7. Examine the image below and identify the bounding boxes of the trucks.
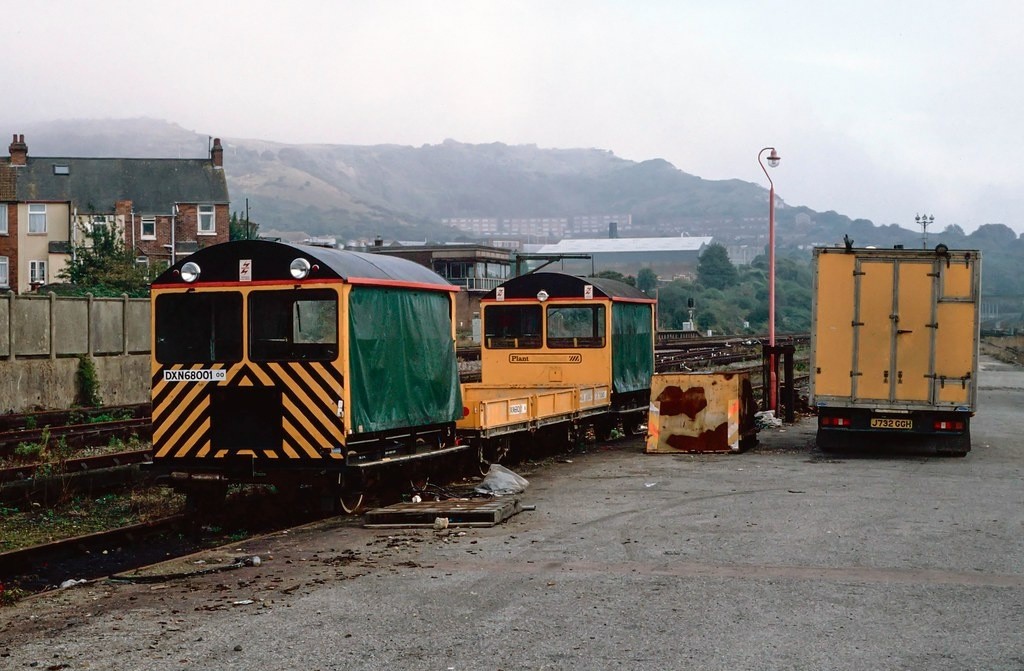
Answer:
[809,245,982,457]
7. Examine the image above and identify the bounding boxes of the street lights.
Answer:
[915,212,937,249]
[756,145,780,414]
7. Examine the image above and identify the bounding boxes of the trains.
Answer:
[144,238,659,516]
